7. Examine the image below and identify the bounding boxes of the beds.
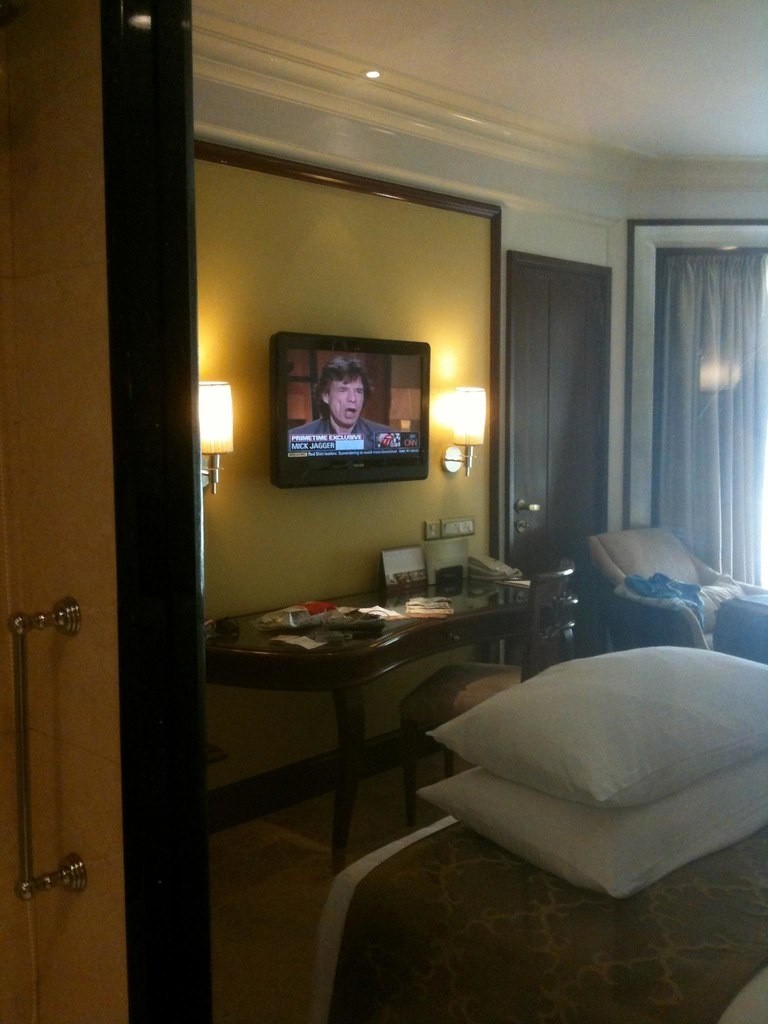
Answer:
[310,818,768,1024]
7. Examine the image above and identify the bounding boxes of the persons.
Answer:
[287,358,396,452]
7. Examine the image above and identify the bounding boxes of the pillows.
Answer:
[420,643,768,810]
[418,753,768,900]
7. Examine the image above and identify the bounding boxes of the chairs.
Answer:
[400,556,577,828]
[585,524,767,652]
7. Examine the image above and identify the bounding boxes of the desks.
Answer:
[206,572,578,877]
[712,593,768,665]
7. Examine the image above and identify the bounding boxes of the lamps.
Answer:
[442,387,487,477]
[199,381,234,495]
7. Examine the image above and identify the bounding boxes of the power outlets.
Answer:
[441,515,475,537]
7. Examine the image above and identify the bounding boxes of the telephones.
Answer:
[468,554,511,577]
